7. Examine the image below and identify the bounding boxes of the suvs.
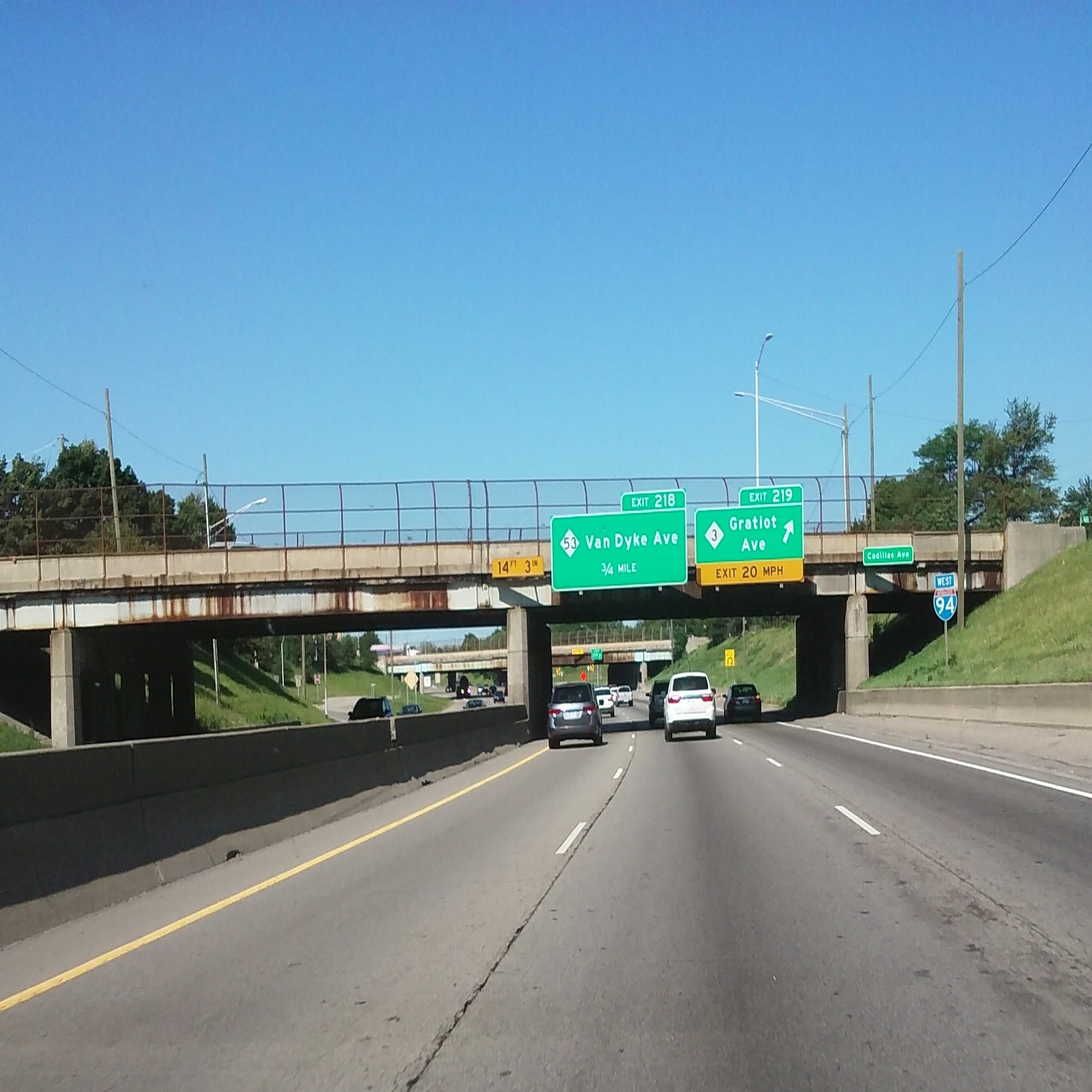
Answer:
[722,684,762,722]
[347,695,393,723]
[645,678,670,725]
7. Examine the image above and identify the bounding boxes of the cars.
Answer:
[396,704,424,718]
[457,686,472,699]
[444,687,453,693]
[463,700,487,711]
[475,684,506,704]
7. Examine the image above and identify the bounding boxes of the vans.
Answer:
[594,683,634,719]
[663,671,717,741]
[546,682,604,748]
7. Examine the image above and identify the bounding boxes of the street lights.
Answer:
[752,330,778,491]
[735,387,857,529]
[204,495,269,708]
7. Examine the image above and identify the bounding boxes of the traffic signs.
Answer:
[591,648,603,662]
[691,508,809,586]
